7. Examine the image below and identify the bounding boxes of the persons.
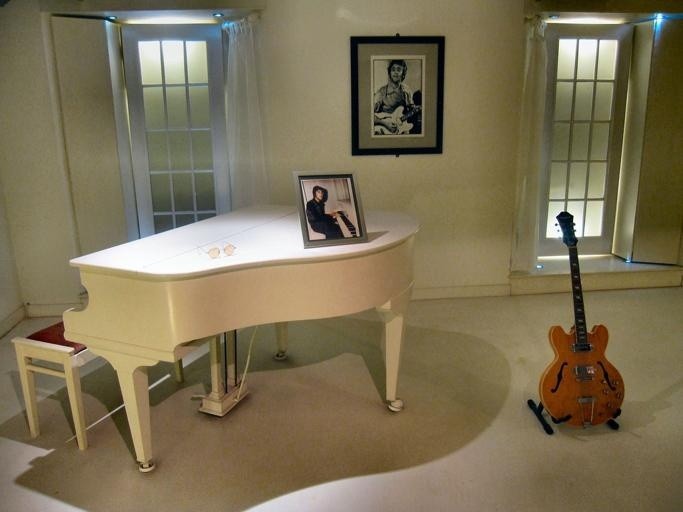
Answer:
[373,59,416,133]
[306,185,343,240]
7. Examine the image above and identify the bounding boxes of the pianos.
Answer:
[62,201,419,472]
[335,210,357,238]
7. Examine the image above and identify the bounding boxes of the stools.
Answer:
[11,321,184,451]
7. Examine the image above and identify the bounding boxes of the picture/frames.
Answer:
[350,35,446,156]
[292,168,369,250]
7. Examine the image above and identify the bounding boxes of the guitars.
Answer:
[538,211,623,428]
[374,106,420,135]
